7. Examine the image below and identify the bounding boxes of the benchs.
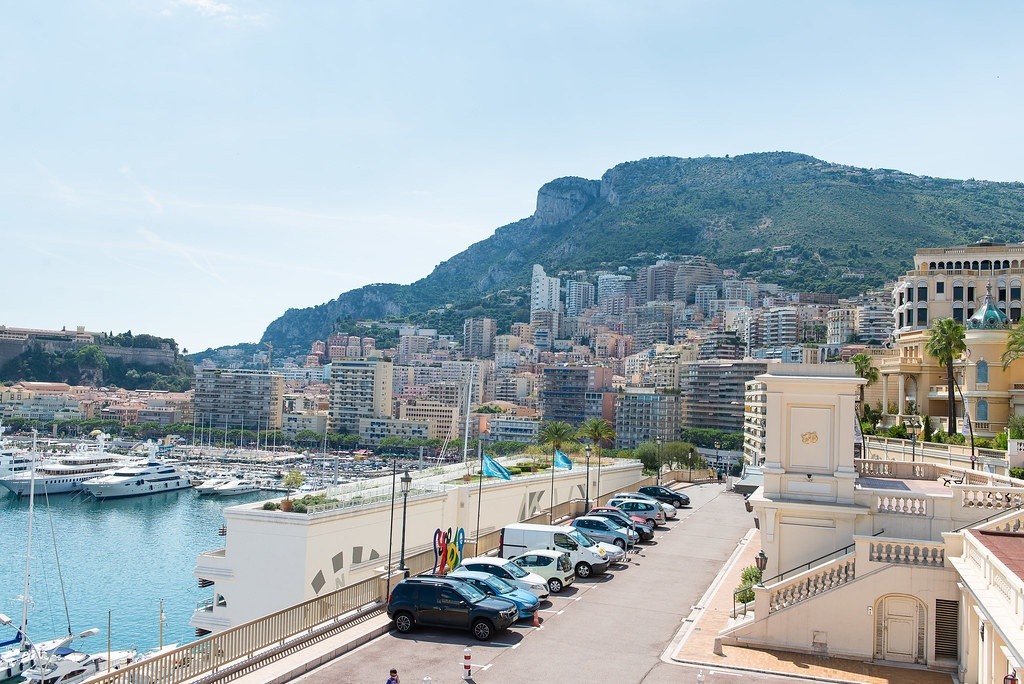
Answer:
[942,470,965,487]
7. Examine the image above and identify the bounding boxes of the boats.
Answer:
[0,434,139,496]
[195,476,261,496]
[81,439,194,498]
[173,413,429,491]
[0,447,54,478]
[0,429,179,684]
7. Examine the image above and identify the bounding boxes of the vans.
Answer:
[498,523,611,578]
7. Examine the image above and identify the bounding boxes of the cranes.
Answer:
[263,341,274,373]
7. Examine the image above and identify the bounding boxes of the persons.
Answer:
[385,668,400,684]
[709,467,715,484]
[716,466,724,485]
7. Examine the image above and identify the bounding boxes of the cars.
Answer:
[571,516,639,549]
[420,569,540,620]
[603,499,667,529]
[586,506,655,542]
[639,486,690,508]
[612,492,676,519]
[511,549,576,593]
[460,557,550,602]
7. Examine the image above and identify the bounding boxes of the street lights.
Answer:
[585,445,593,514]
[0,613,101,684]
[714,441,720,468]
[399,469,412,568]
[655,435,662,486]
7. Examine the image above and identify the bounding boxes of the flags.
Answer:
[554,448,572,470]
[483,453,512,480]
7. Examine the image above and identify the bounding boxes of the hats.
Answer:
[390,668,397,677]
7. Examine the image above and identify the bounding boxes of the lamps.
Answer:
[744,494,753,513]
[755,549,769,571]
[807,473,812,479]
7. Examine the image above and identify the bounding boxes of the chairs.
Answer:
[587,521,596,529]
[596,521,600,530]
[634,505,639,509]
[630,504,634,509]
[654,488,661,494]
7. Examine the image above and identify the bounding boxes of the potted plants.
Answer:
[540,443,552,463]
[279,470,306,512]
[463,457,475,484]
[525,444,542,472]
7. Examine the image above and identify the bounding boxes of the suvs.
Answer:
[387,574,519,641]
